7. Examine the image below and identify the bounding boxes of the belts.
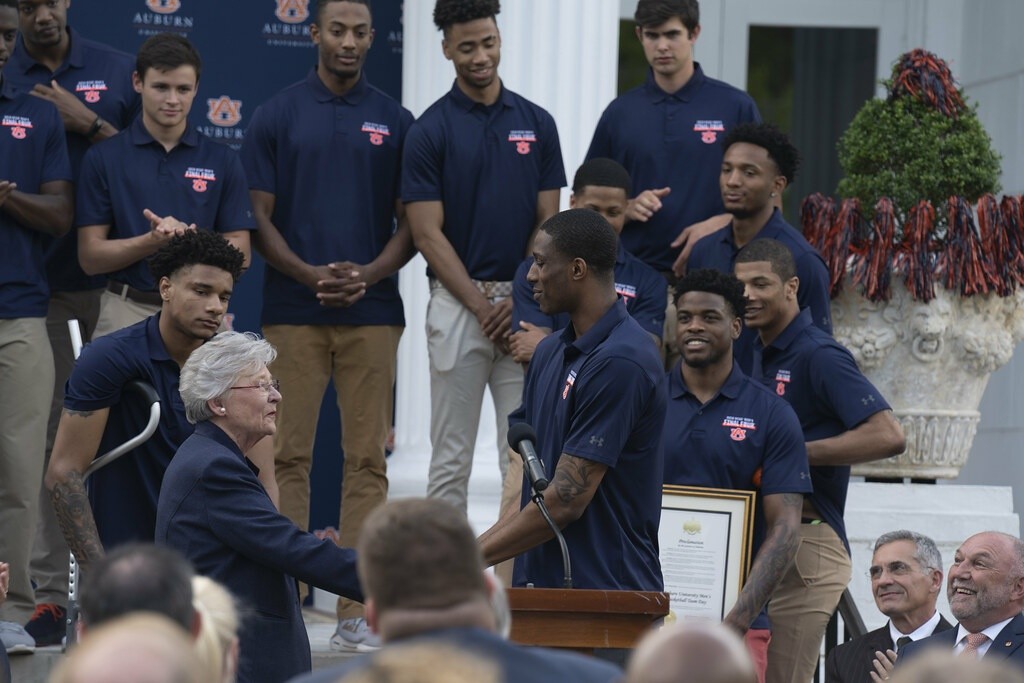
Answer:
[430,278,513,298]
[107,280,163,307]
[801,518,827,525]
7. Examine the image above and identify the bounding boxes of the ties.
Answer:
[896,636,914,655]
[958,633,990,661]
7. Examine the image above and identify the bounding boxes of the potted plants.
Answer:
[808,51,1024,477]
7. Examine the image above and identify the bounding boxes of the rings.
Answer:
[884,677,891,681]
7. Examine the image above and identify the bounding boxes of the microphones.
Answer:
[507,422,548,491]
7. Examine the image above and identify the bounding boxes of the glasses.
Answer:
[229,379,279,392]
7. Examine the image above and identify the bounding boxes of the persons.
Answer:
[0,0,833,650]
[823,530,956,683]
[894,531,1024,683]
[477,123,907,683]
[1,499,760,682]
[52,230,369,683]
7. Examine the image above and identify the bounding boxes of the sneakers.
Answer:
[329,617,382,652]
[0,621,36,653]
[25,604,67,652]
